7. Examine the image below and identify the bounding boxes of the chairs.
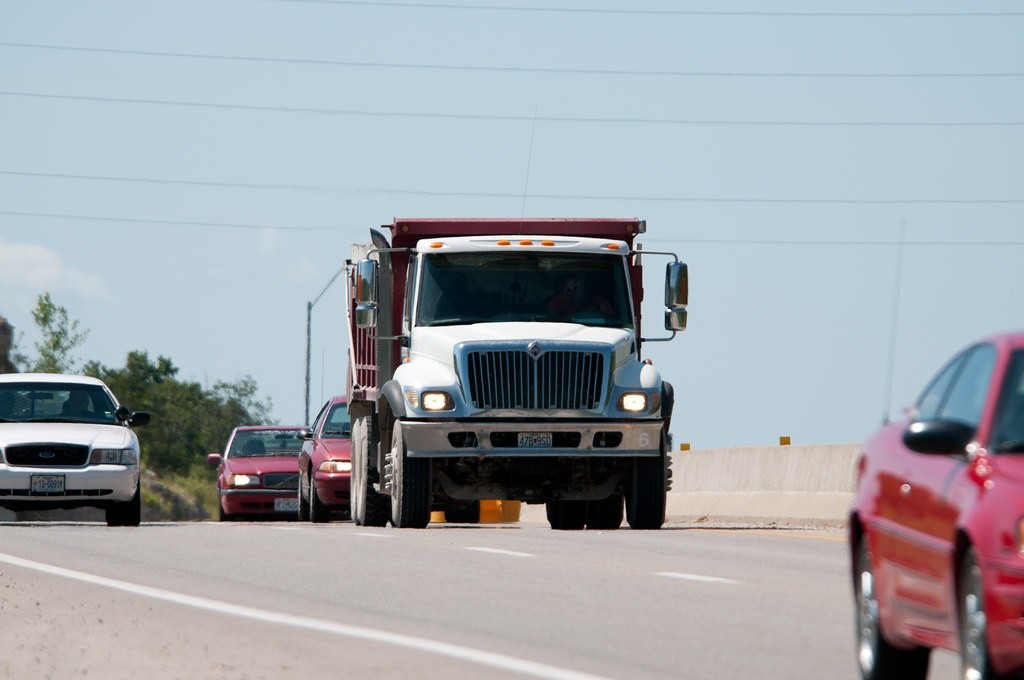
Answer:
[62,400,73,416]
[247,439,266,455]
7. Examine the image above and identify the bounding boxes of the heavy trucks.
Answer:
[344,216,689,529]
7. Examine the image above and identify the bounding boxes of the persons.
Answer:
[67,390,95,420]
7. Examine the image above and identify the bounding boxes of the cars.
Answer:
[208,426,314,521]
[846,335,1024,679]
[296,396,352,523]
[0,373,152,527]
